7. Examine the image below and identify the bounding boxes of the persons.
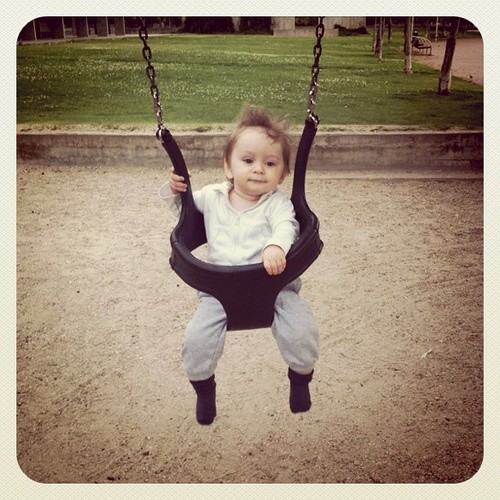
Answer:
[162,104,318,425]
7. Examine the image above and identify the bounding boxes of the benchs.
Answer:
[412,37,433,55]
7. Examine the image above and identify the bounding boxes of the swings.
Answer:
[136,17,324,331]
